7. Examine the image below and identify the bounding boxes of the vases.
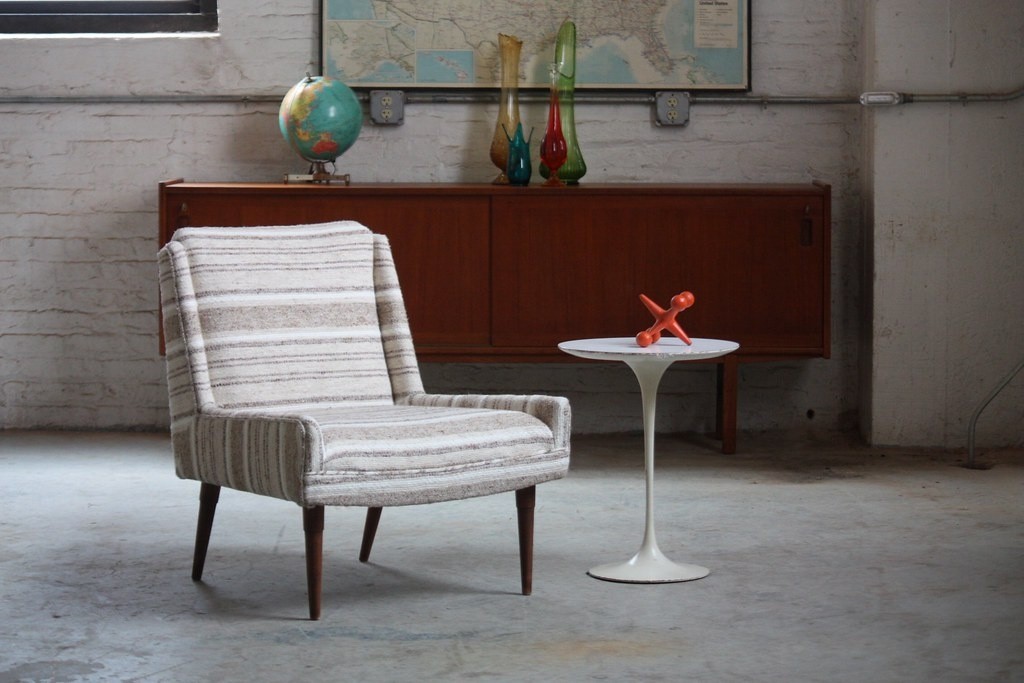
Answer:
[539,21,587,183]
[501,121,534,186]
[489,32,523,184]
[539,62,568,186]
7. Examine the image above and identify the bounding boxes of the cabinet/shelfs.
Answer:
[158,178,832,455]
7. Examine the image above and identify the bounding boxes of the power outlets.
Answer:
[368,90,404,126]
[654,91,691,126]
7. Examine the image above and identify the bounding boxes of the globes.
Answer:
[278,70,364,186]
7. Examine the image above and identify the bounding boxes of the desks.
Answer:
[557,338,740,584]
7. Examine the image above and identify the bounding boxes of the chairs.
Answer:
[157,221,571,620]
